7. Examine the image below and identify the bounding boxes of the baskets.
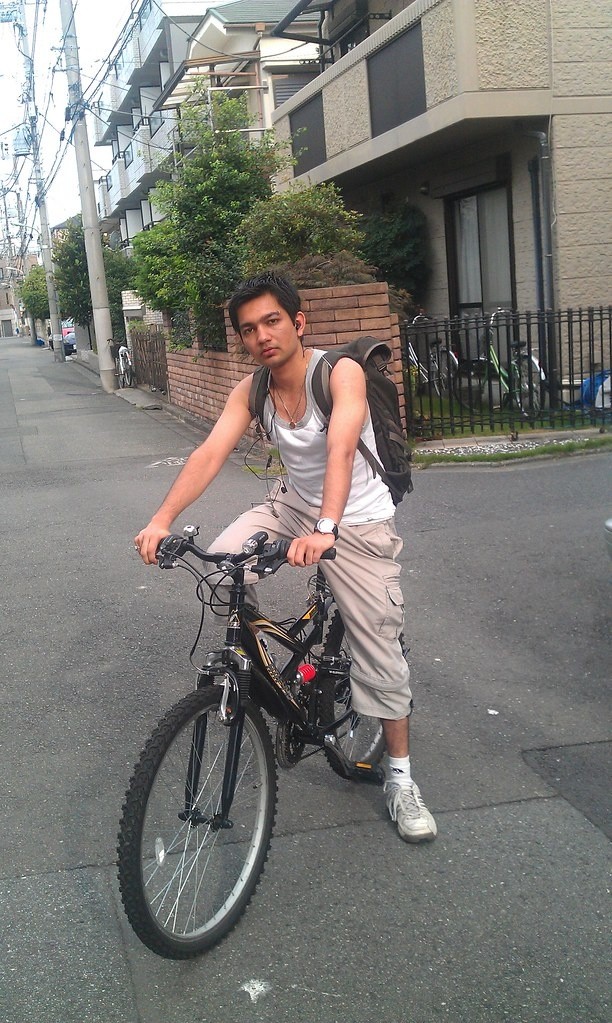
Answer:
[110,345,125,357]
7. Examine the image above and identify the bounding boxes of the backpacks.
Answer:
[248,336,415,507]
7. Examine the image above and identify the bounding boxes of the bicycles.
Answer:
[454,308,547,422]
[113,524,403,963]
[398,314,459,403]
[109,335,134,389]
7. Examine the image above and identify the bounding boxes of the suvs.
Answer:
[48,317,76,350]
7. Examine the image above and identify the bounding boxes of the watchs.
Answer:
[314,519,340,540]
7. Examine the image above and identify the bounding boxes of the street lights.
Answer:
[0,283,24,338]
[10,222,67,362]
[6,267,40,346]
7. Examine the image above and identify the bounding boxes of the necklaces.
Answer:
[275,354,307,429]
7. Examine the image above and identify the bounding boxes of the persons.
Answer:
[135,274,438,842]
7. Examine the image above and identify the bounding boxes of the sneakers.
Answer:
[383,781,437,843]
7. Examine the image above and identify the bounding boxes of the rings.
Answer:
[135,546,140,551]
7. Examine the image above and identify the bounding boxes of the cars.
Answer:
[63,331,76,356]
